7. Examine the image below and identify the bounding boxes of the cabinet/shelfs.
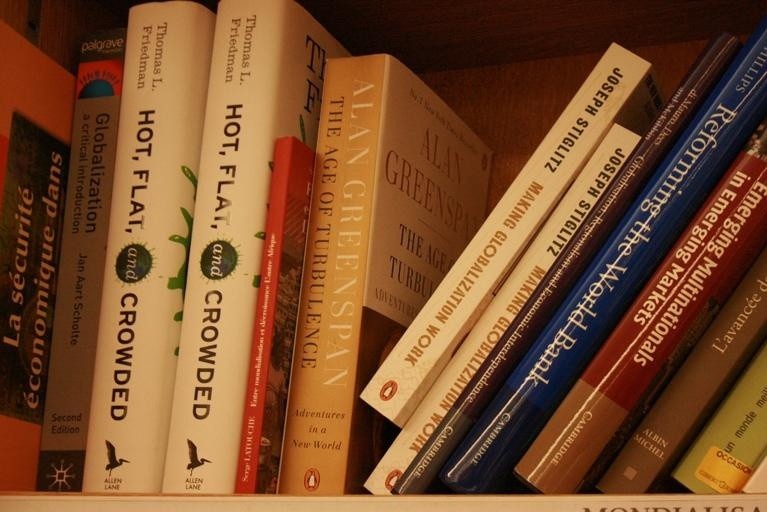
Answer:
[3,3,767,512]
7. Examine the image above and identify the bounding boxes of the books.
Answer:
[2,2,767,493]
